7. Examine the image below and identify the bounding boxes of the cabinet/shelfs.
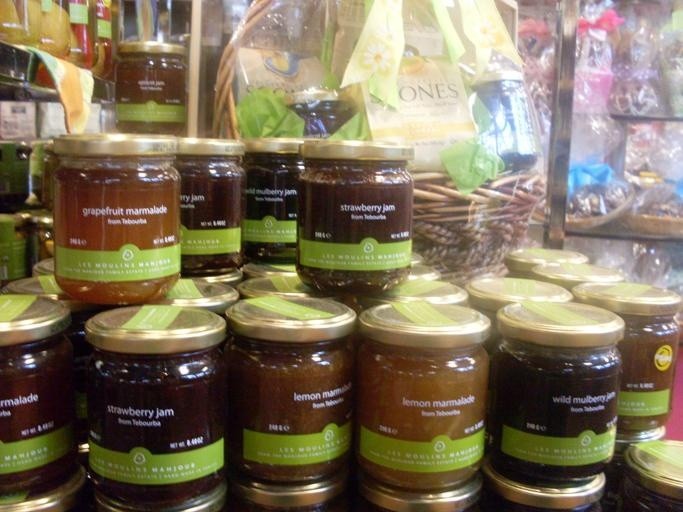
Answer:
[566,112,683,242]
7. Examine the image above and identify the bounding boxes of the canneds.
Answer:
[285,90,351,135]
[119,41,186,132]
[470,70,538,167]
[51,137,182,306]
[0,249,683,512]
[295,141,415,296]
[0,80,112,137]
[178,138,246,277]
[242,138,305,264]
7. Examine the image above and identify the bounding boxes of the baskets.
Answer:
[563,179,638,232]
[212,1,549,281]
[584,212,682,239]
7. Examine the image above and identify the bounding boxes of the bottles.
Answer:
[0,0,114,80]
